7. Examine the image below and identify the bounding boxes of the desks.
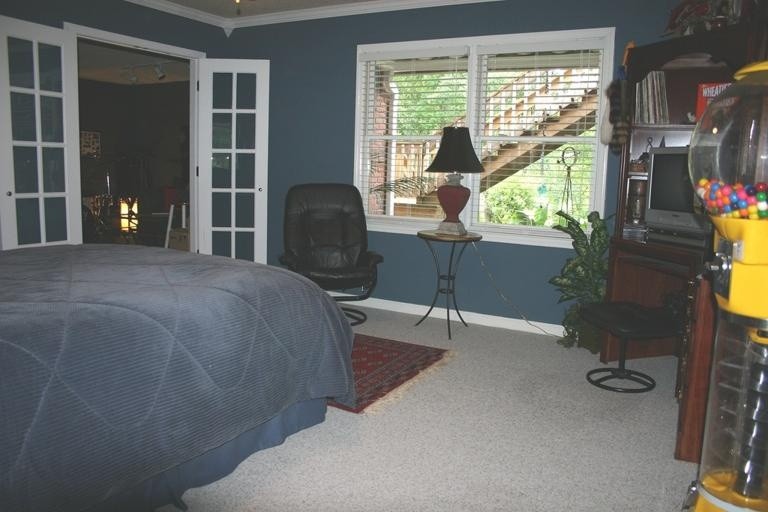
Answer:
[416,229,483,339]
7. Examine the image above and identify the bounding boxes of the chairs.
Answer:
[278,183,384,325]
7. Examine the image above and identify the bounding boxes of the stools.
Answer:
[580,302,667,392]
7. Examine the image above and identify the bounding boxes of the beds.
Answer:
[0,242,331,512]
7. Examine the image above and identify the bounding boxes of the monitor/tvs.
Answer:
[645,146,718,234]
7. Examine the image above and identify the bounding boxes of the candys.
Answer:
[695,178,768,219]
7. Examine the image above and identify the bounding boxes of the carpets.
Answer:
[328,332,448,414]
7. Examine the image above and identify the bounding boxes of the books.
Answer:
[634,70,669,124]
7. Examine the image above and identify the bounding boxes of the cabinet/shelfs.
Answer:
[605,11,768,466]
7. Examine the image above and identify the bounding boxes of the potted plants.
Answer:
[551,209,617,354]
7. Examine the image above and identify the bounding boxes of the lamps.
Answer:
[424,122,485,237]
